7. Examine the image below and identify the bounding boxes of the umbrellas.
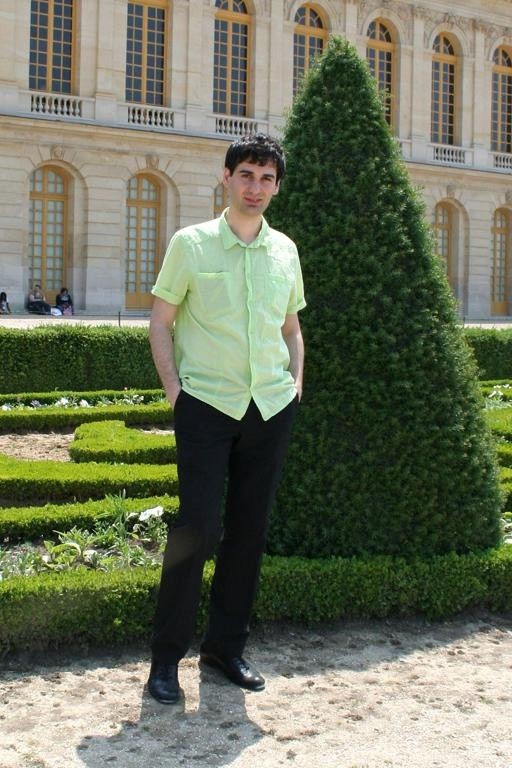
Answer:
[198,651,265,691]
[148,659,179,705]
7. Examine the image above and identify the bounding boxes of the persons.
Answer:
[28,285,50,315]
[148,132,307,705]
[56,288,72,313]
[0,292,11,315]
